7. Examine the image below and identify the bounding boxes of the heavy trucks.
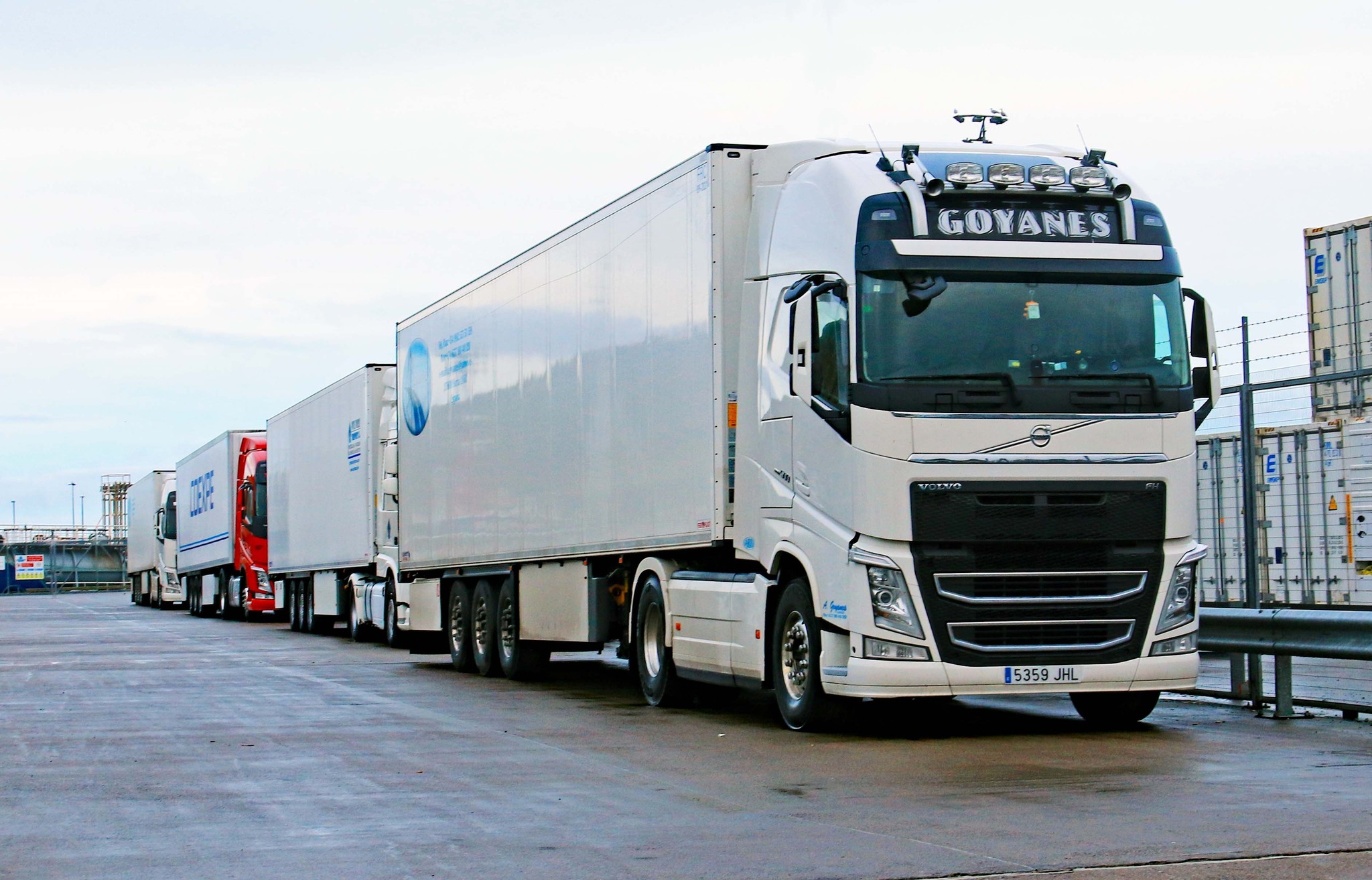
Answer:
[266,363,402,647]
[394,108,1221,730]
[175,430,290,622]
[127,470,188,610]
